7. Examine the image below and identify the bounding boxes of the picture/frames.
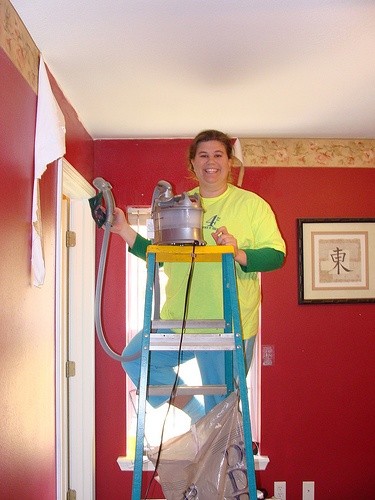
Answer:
[296,216,375,306]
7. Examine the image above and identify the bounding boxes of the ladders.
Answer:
[132,245,257,500]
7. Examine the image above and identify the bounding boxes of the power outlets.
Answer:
[274,481,286,500]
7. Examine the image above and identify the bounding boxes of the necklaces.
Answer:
[200,193,205,198]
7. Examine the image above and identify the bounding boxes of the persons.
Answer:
[101,130,286,427]
